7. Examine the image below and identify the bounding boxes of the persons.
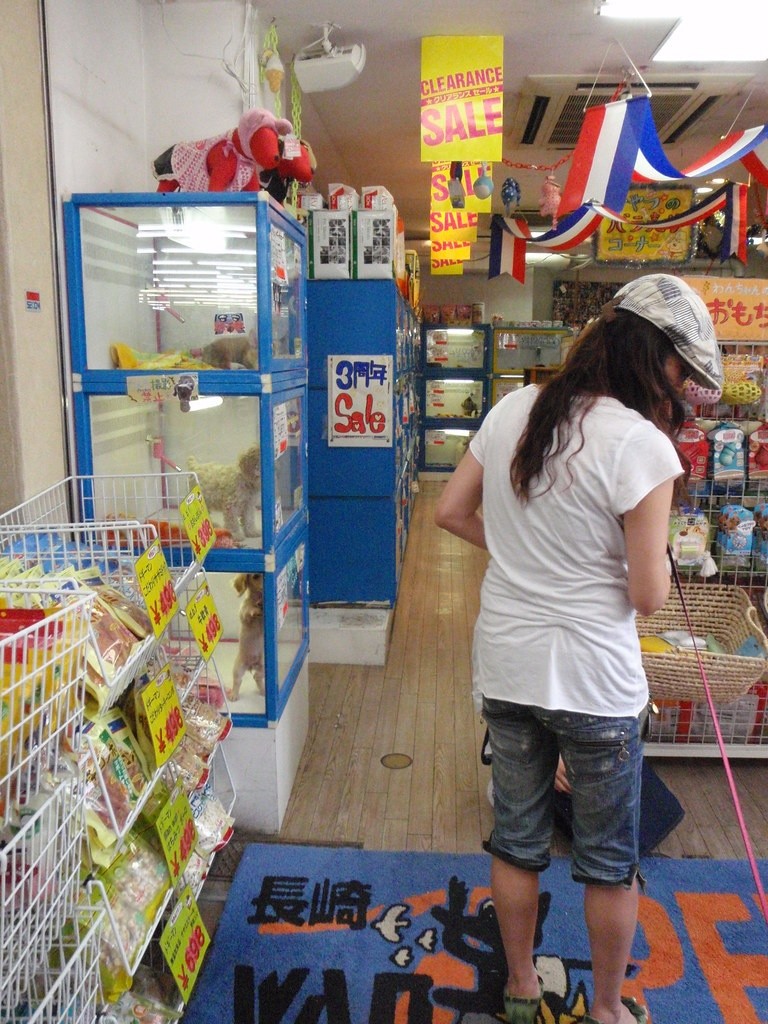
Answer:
[435,273,722,1024]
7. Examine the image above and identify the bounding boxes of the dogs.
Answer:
[186,443,262,544]
[228,573,267,703]
[198,326,257,369]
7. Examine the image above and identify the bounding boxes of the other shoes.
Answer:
[584,996,648,1024]
[503,971,544,1024]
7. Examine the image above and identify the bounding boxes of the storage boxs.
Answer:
[348,209,397,279]
[296,193,326,210]
[306,209,350,279]
[328,183,359,210]
[361,185,394,210]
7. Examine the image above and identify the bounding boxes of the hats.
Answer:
[600,273,724,389]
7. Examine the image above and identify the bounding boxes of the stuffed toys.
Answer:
[149,107,317,206]
[539,179,560,218]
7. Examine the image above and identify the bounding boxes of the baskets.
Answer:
[635,583,768,705]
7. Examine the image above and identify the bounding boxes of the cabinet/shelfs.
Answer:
[305,278,422,608]
[421,323,495,473]
[488,326,571,410]
[59,191,312,835]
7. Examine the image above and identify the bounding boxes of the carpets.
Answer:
[177,843,768,1024]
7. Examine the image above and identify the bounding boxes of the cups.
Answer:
[472,302,485,323]
